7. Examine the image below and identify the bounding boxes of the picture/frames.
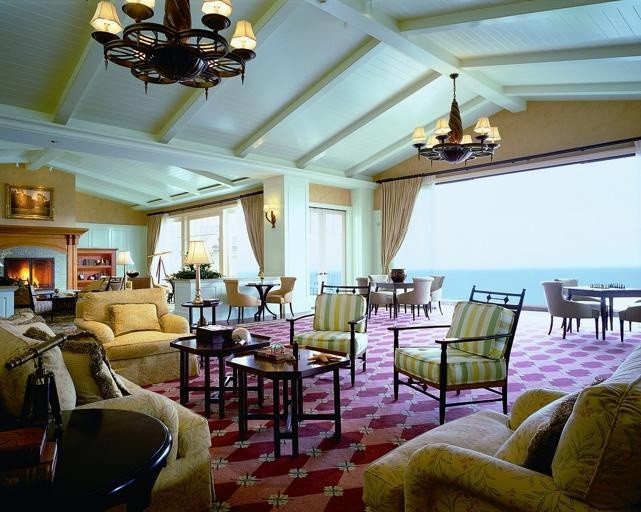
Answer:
[5,182,56,222]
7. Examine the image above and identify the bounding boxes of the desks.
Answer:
[2,407,173,512]
[182,301,222,333]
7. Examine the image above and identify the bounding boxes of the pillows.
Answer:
[107,302,163,337]
[493,371,614,474]
[22,325,133,405]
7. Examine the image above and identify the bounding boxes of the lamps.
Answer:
[410,72,503,168]
[89,0,259,101]
[115,249,135,290]
[184,241,213,305]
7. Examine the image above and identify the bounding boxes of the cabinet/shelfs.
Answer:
[77,248,119,283]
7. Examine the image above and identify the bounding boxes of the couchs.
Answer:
[2,308,218,511]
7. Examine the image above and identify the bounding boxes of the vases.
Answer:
[391,268,407,283]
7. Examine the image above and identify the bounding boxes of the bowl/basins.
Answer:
[231,328,251,342]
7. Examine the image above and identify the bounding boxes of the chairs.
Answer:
[541,277,641,344]
[388,285,526,425]
[74,286,199,387]
[223,277,297,322]
[362,346,641,510]
[284,280,372,388]
[355,274,446,323]
[151,275,173,304]
[28,276,111,321]
